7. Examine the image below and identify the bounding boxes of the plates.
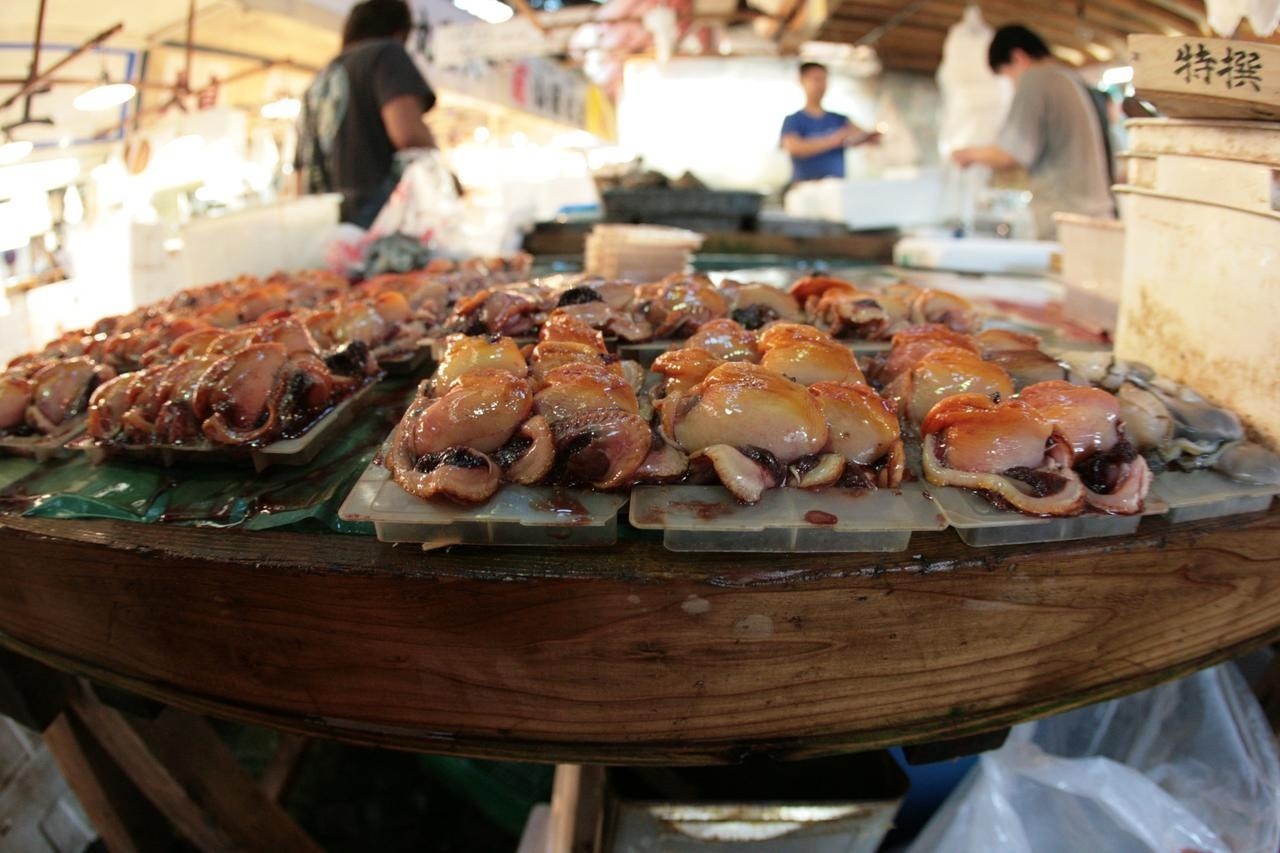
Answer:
[583,221,706,287]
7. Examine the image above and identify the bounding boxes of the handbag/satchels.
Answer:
[338,151,524,278]
[912,661,1280,851]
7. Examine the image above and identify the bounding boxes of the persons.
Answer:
[293,1,462,252]
[781,60,886,193]
[950,21,1123,245]
